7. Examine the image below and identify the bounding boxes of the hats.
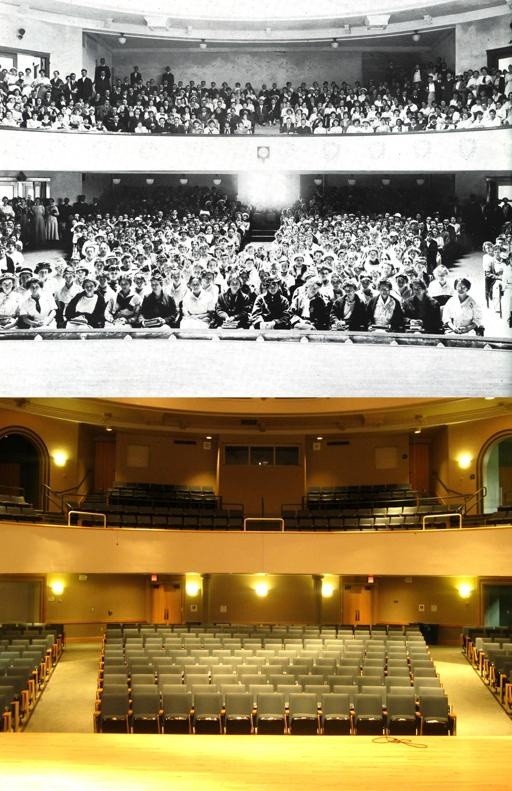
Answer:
[34,261,53,275]
[54,197,423,290]
[0,272,17,285]
[24,277,45,290]
[18,268,34,278]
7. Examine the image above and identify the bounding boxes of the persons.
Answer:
[0,180,512,344]
[0,56,511,136]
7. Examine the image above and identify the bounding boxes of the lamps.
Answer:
[117,33,127,45]
[412,30,421,43]
[200,39,207,51]
[331,38,339,49]
[113,174,223,189]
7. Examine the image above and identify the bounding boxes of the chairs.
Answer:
[0,623,512,736]
[0,481,511,531]
